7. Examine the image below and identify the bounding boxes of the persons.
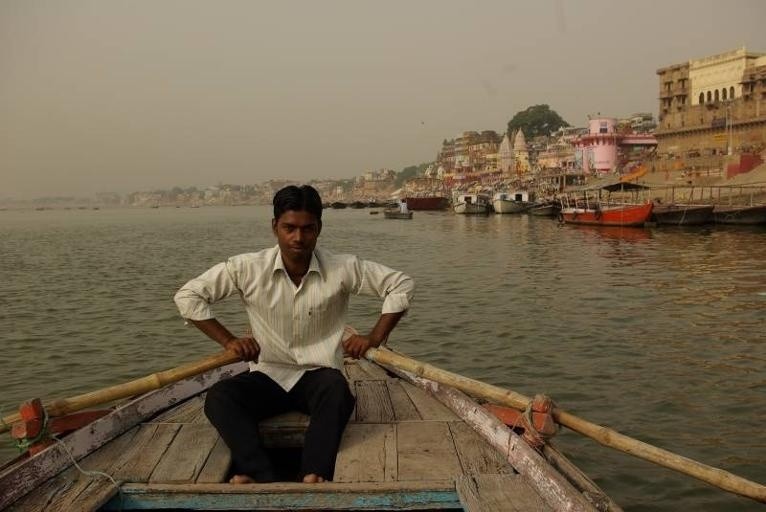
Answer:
[399,200,409,213]
[173,183,415,484]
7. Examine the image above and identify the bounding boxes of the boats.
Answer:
[402,195,451,210]
[0,322,766,512]
[707,198,766,226]
[559,192,656,229]
[528,199,562,220]
[654,200,715,227]
[383,204,414,220]
[454,195,492,213]
[320,201,397,209]
[492,196,535,213]
